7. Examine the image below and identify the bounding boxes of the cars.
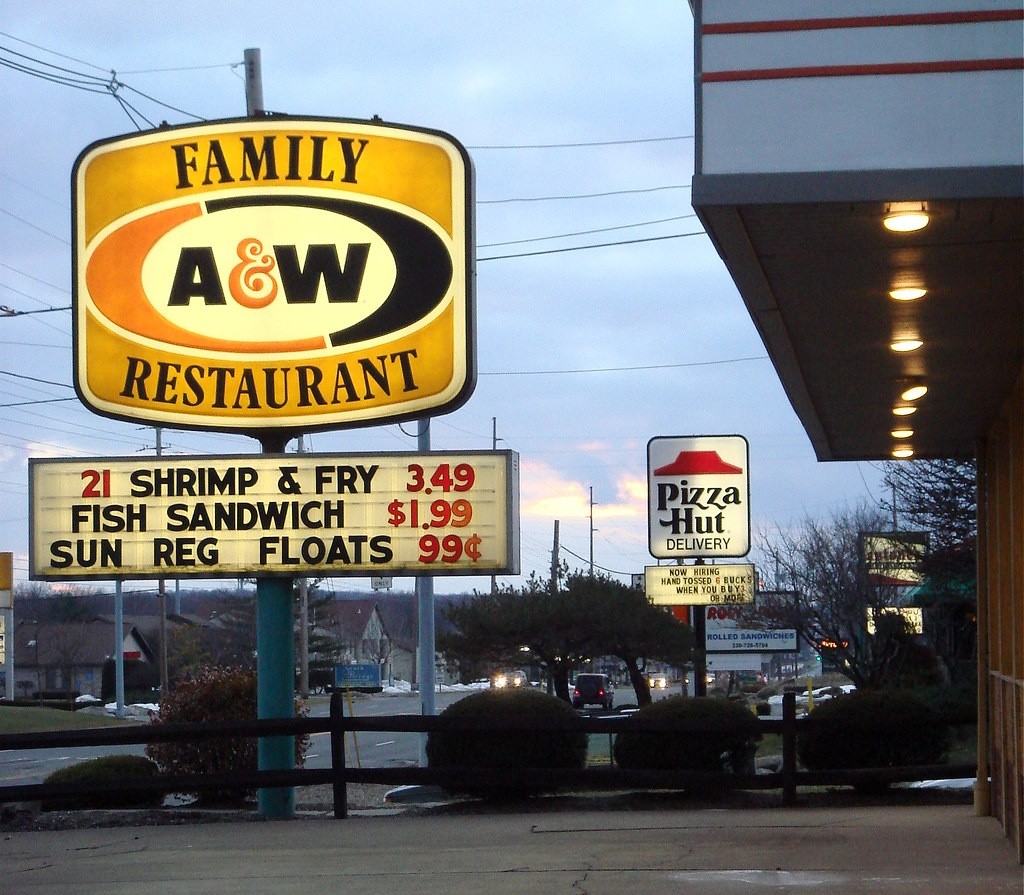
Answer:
[707,673,716,685]
[650,674,667,687]
[492,670,529,689]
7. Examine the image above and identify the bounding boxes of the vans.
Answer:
[573,673,614,711]
